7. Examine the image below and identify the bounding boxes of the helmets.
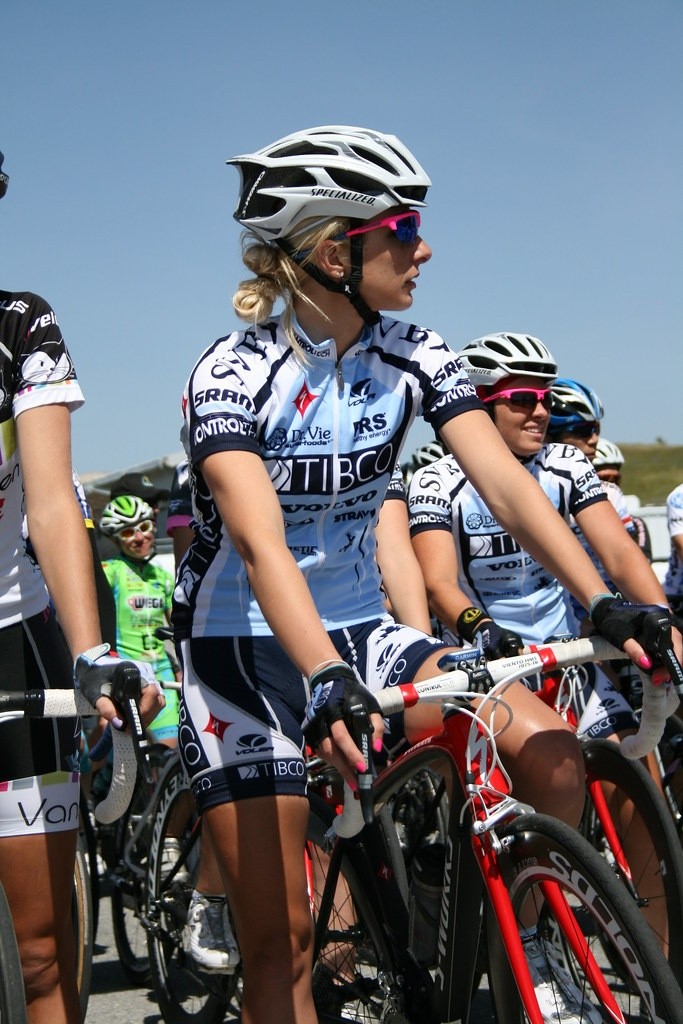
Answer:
[226,125,432,238]
[592,439,625,465]
[549,376,603,427]
[100,496,156,537]
[457,332,559,387]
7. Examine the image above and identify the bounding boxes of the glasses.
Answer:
[481,390,553,410]
[294,211,421,260]
[598,474,622,485]
[547,420,600,438]
[112,519,156,540]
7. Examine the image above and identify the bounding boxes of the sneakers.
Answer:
[521,933,592,1024]
[182,888,239,969]
[160,836,191,884]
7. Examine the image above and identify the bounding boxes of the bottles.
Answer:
[408,841,445,972]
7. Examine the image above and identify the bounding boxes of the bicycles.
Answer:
[66,631,682,1024]
[0,661,154,1023]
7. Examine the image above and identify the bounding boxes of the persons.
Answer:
[83,331,682,977]
[1,141,166,1024]
[166,123,673,1024]
